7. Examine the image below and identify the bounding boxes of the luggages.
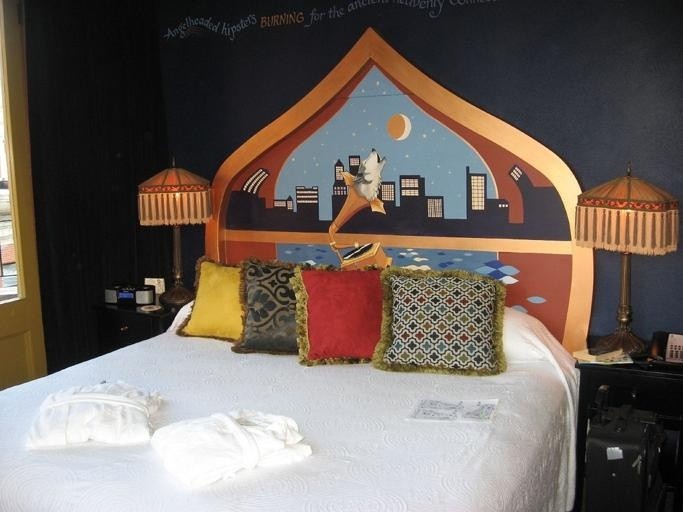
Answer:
[582,384,669,511]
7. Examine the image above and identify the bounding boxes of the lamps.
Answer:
[138,159,209,308]
[571,160,678,360]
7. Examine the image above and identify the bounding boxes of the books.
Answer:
[0,175,17,296]
[573,347,635,365]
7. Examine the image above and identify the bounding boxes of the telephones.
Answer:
[649,331,683,368]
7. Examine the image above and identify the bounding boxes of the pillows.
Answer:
[229,256,304,357]
[286,263,381,366]
[178,253,246,346]
[368,265,507,378]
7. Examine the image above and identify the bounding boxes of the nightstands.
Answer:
[93,291,168,339]
[568,360,679,508]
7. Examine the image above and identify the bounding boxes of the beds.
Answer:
[0,28,605,510]
